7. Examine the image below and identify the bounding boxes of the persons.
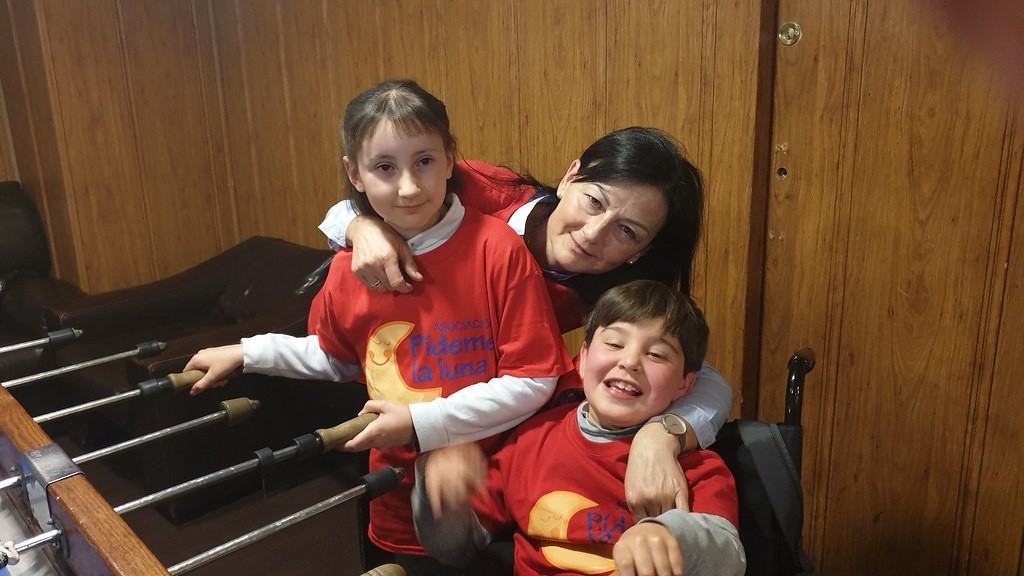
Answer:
[181,79,575,576]
[317,126,736,524]
[410,279,746,575]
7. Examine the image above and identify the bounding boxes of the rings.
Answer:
[371,280,382,288]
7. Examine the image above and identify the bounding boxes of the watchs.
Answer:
[644,413,686,454]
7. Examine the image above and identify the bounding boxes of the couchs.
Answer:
[0,181,92,441]
[42,235,369,523]
